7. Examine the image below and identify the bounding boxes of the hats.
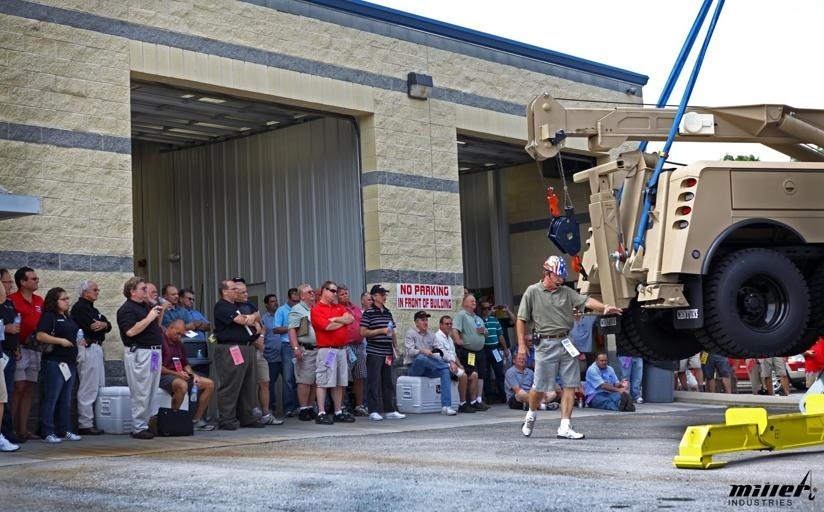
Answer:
[370,285,390,295]
[414,311,432,320]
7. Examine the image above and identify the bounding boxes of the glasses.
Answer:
[90,288,101,292]
[28,277,40,281]
[328,288,337,294]
[188,297,195,301]
[59,297,69,301]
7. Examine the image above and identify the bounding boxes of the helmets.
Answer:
[542,254,568,278]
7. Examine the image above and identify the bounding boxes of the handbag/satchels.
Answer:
[22,327,55,355]
[158,407,195,437]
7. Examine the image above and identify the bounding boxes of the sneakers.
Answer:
[557,424,585,440]
[260,414,284,425]
[131,429,155,439]
[298,405,370,425]
[521,412,537,438]
[458,399,492,414]
[441,406,457,416]
[192,416,237,432]
[541,401,560,411]
[385,410,407,420]
[239,418,265,428]
[367,411,383,421]
[9,426,104,444]
[0,434,21,453]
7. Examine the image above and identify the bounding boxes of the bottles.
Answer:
[11,314,21,334]
[475,319,481,333]
[189,383,198,402]
[158,296,172,311]
[76,328,84,346]
[196,349,203,359]
[577,397,582,408]
[386,320,393,337]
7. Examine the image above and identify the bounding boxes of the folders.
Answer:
[296,316,309,337]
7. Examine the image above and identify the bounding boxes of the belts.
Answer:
[317,346,348,350]
[122,345,163,350]
[88,340,104,346]
[538,331,571,340]
[296,341,317,349]
[216,341,256,347]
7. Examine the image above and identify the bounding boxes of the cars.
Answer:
[726,353,806,395]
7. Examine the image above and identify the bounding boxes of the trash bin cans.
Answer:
[641,360,676,403]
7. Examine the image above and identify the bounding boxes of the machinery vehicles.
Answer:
[525,92,824,364]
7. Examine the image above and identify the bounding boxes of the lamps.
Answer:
[406,70,433,101]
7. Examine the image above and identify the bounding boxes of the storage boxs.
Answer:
[394,374,463,416]
[94,385,192,436]
